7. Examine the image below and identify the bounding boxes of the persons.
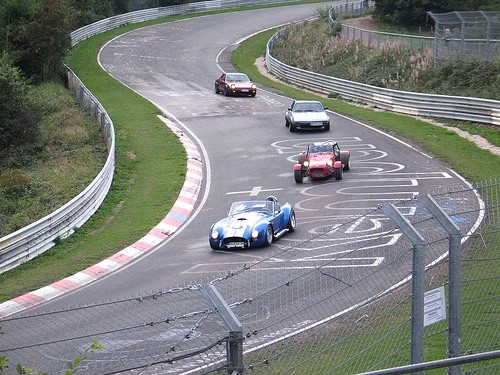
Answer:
[441,29,453,56]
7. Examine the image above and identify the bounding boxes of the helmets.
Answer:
[266,195,277,208]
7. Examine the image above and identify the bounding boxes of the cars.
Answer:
[284,99,331,133]
[214,72,257,97]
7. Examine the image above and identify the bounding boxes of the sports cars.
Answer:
[209,196,296,251]
[294,139,351,183]
